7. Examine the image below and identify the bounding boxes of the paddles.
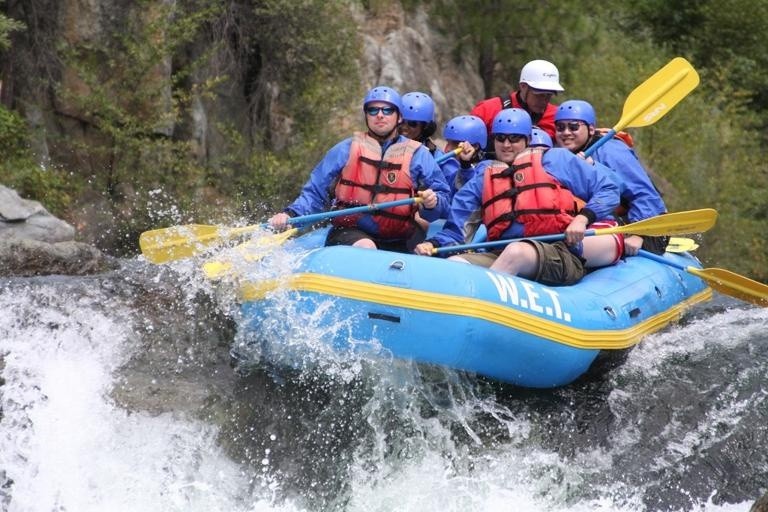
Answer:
[580,56,699,161]
[430,209,717,252]
[139,196,422,265]
[638,250,768,310]
[200,226,308,279]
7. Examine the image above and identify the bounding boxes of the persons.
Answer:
[268,85,451,255]
[415,108,621,286]
[400,59,670,265]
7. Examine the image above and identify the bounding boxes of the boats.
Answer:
[171,222,714,388]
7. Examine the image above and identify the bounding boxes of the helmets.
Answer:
[363,86,597,148]
[519,60,564,91]
